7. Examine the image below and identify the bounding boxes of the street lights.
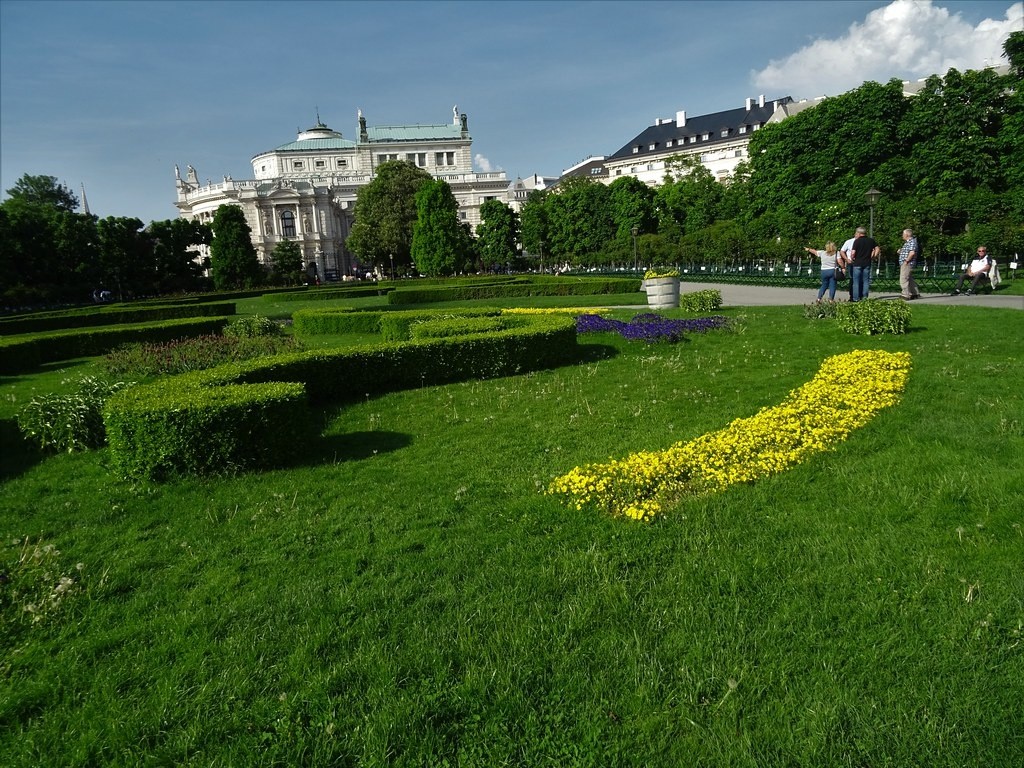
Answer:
[629,225,638,278]
[537,240,544,272]
[388,254,395,279]
[862,185,883,239]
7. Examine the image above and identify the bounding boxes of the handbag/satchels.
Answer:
[834,267,845,281]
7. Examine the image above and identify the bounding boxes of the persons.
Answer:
[951,244,992,296]
[851,227,880,302]
[840,227,856,302]
[897,229,918,301]
[804,241,846,303]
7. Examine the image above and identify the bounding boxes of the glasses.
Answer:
[978,250,985,252]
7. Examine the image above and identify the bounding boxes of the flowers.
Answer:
[644,267,680,280]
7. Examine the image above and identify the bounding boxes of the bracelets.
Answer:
[842,268,845,269]
[808,248,810,251]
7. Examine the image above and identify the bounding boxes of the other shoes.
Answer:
[951,290,960,296]
[815,299,821,306]
[964,290,972,296]
[828,299,833,305]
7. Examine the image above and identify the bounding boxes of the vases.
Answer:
[646,277,680,309]
